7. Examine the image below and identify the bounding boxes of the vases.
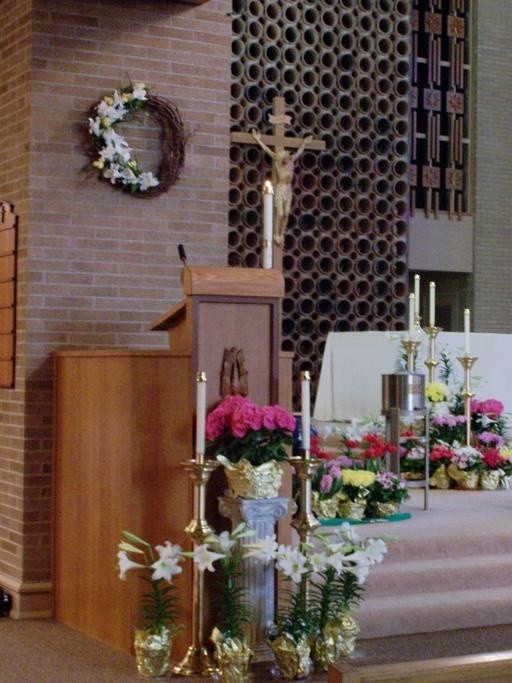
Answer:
[215,454,285,500]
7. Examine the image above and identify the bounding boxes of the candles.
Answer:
[408,274,471,355]
[195,371,207,455]
[261,181,274,269]
[301,370,310,450]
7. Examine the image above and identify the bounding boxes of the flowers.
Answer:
[205,395,297,466]
[310,335,512,519]
[85,81,185,199]
